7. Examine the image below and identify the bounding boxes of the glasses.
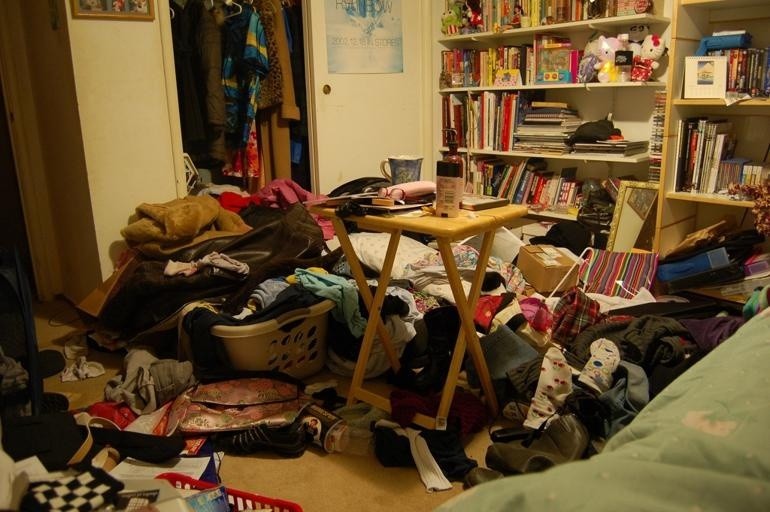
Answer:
[377,187,408,205]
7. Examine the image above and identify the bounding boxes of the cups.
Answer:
[379,153,424,187]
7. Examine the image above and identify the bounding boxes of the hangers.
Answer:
[208,1,292,19]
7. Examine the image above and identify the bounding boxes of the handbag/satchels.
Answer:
[575,242,661,305]
[656,213,767,294]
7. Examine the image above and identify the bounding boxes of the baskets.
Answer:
[145,471,303,512]
[211,298,337,380]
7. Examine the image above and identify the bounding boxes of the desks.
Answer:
[306,199,528,430]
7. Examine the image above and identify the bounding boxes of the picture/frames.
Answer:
[70,1,155,20]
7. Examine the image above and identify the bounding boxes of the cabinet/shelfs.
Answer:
[436,0,770,305]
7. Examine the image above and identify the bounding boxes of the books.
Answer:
[300,186,379,202]
[355,198,433,213]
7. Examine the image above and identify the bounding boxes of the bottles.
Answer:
[435,158,465,218]
